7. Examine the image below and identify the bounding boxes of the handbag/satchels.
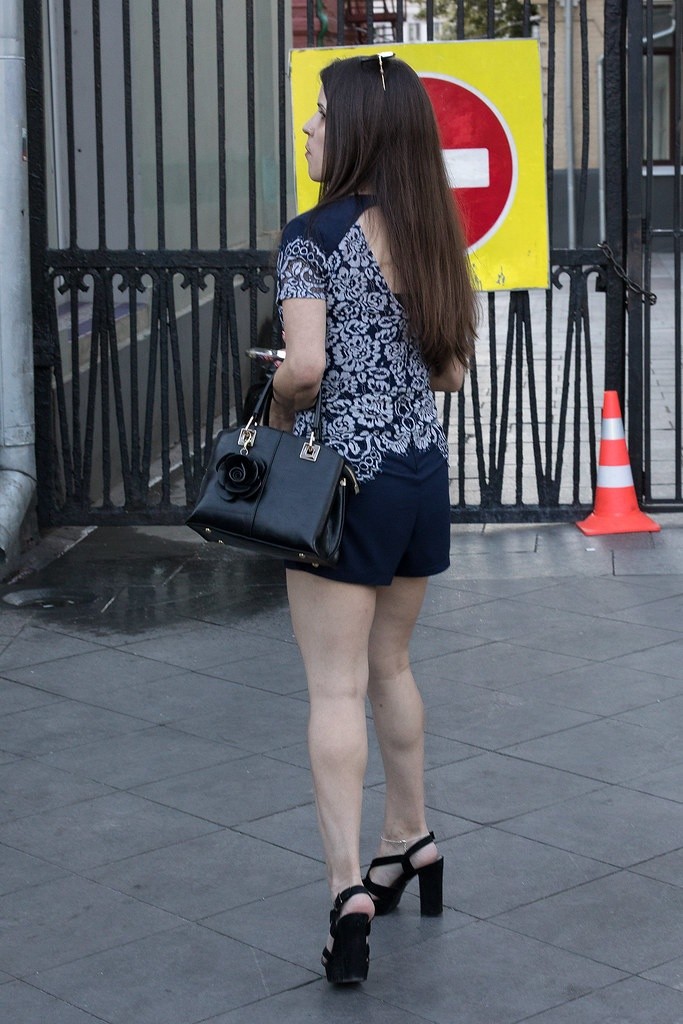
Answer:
[184,373,359,570]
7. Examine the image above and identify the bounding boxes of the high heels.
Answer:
[361,831,444,916]
[321,885,376,983]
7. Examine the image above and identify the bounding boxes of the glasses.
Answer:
[377,51,394,92]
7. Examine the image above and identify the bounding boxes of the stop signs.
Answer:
[282,36,553,294]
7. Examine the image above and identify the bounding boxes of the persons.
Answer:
[266,55,478,984]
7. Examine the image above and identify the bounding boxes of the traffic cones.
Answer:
[573,390,663,539]
